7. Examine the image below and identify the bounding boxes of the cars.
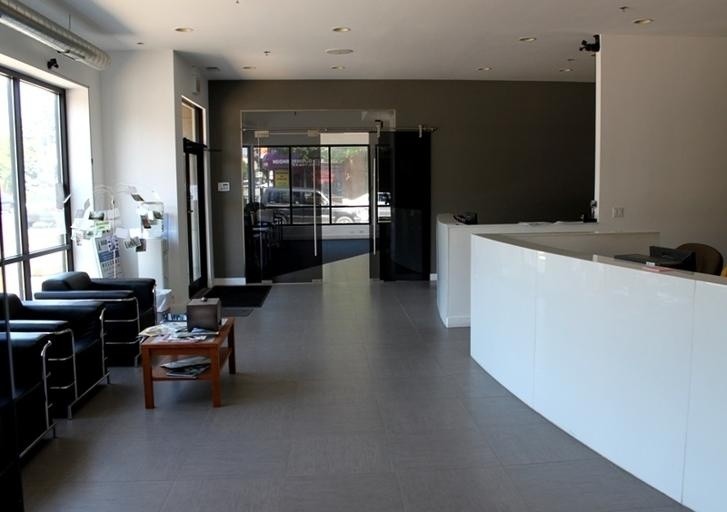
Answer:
[350,192,391,221]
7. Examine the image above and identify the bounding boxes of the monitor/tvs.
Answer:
[649,245,696,271]
[464,211,478,224]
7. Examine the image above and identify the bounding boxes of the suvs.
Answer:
[259,188,359,224]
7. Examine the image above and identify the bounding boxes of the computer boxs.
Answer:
[614,254,682,270]
[454,214,470,224]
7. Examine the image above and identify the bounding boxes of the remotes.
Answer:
[192,331,221,337]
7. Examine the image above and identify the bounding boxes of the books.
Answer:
[135,318,227,380]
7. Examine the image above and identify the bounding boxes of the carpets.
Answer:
[204,284,273,308]
[222,308,253,317]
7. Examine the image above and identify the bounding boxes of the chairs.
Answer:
[0,332,57,467]
[676,242,723,276]
[33,271,157,368]
[249,208,283,265]
[0,292,111,420]
